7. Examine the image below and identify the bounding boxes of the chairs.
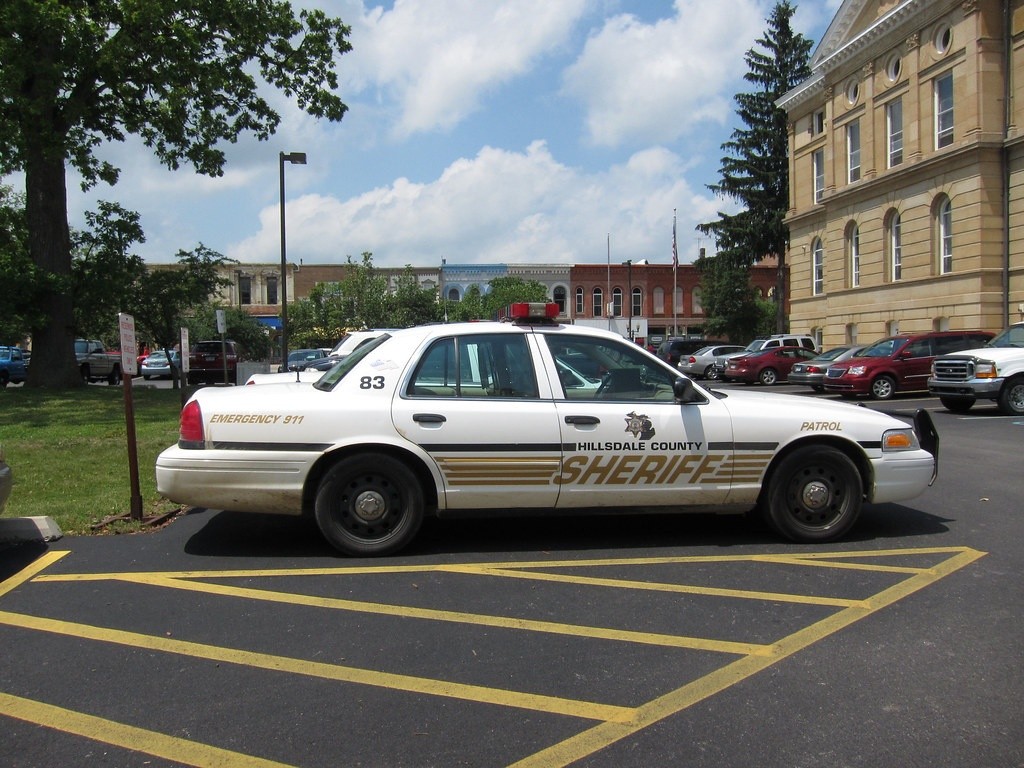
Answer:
[503,342,538,397]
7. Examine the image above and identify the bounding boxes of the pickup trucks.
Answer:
[73,338,123,386]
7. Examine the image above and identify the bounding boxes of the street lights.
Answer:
[621,260,633,340]
[233,268,249,342]
[278,151,309,374]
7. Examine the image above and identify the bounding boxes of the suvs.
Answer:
[653,335,739,370]
[926,320,1024,417]
[711,333,820,380]
[822,329,1008,401]
[0,344,32,387]
[185,340,243,386]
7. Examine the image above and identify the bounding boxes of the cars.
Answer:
[724,345,821,387]
[677,344,753,380]
[787,343,893,393]
[141,349,183,381]
[150,302,940,560]
[242,325,650,390]
